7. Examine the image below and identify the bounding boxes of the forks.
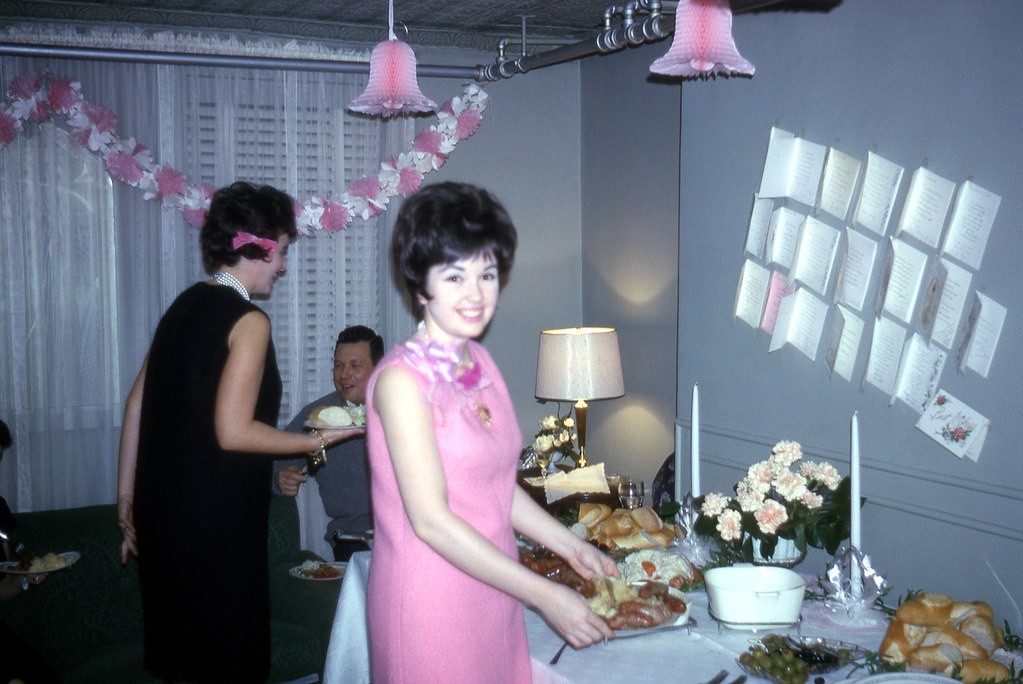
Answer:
[0,530,33,560]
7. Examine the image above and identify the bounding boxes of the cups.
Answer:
[619,479,646,510]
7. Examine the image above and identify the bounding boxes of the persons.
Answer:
[367,181,619,684]
[117,184,364,684]
[272,326,384,562]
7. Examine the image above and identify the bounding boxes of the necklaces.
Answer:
[209,272,250,301]
[418,321,493,426]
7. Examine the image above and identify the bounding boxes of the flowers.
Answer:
[0,69,489,251]
[532,415,581,464]
[690,441,867,562]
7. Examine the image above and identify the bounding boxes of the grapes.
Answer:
[740,636,850,684]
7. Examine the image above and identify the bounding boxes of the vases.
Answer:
[751,535,800,564]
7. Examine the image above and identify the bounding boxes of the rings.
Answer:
[121,527,129,532]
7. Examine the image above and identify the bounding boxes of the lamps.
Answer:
[347,0,437,116]
[535,326,626,466]
[692,382,700,497]
[850,411,861,593]
[650,0,755,76]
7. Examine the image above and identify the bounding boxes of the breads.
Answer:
[568,504,677,550]
[314,564,340,578]
[309,404,353,428]
[880,592,1011,684]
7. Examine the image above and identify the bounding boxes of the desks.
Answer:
[323,538,1023,684]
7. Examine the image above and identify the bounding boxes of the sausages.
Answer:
[602,582,688,628]
[519,544,595,596]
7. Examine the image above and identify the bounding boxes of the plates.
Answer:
[303,420,369,430]
[0,552,81,574]
[289,561,348,580]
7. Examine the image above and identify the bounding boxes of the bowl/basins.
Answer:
[705,567,806,631]
[854,673,965,684]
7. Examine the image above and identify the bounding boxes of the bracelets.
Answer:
[308,429,327,465]
